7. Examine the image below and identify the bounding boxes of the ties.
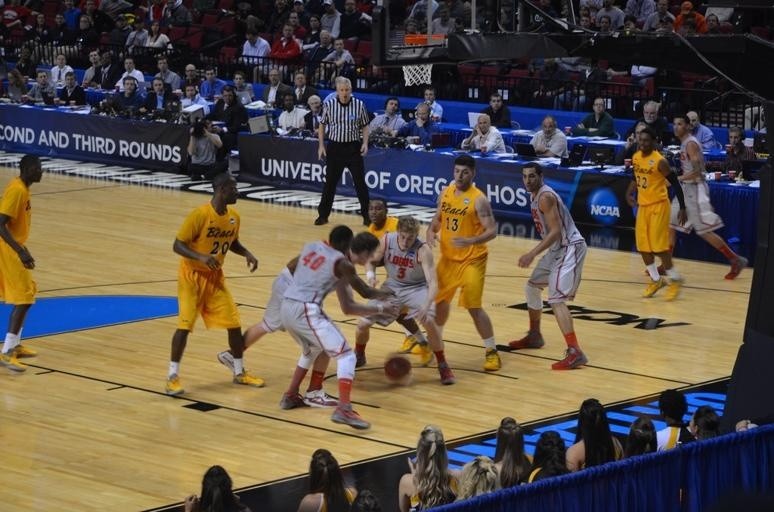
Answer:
[58,70,61,80]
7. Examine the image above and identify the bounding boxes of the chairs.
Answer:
[159,1,374,74]
[456,62,654,108]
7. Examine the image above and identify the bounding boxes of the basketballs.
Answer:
[385,358,411,383]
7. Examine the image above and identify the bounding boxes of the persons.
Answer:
[399,417,573,511]
[644,112,748,280]
[186,464,251,511]
[162,172,267,396]
[2,1,774,228]
[218,156,593,429]
[625,130,690,304]
[656,389,698,452]
[299,449,384,511]
[691,405,761,441]
[621,416,658,458]
[566,398,624,471]
[0,155,44,372]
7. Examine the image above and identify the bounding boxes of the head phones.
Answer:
[414,103,434,119]
[123,76,139,89]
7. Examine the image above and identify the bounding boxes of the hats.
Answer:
[324,0,333,5]
[680,1,692,15]
[293,0,303,4]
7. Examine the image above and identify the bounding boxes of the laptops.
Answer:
[513,142,539,161]
[40,91,54,105]
[424,134,451,150]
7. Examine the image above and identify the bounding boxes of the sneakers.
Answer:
[364,218,371,226]
[315,216,328,225]
[725,256,748,279]
[643,264,681,301]
[0,348,27,372]
[7,344,38,358]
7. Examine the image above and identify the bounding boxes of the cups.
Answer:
[83,81,88,89]
[53,98,58,107]
[115,85,120,93]
[729,170,736,181]
[725,144,731,154]
[481,146,486,155]
[624,158,632,170]
[564,126,572,136]
[715,171,722,181]
[407,135,420,144]
